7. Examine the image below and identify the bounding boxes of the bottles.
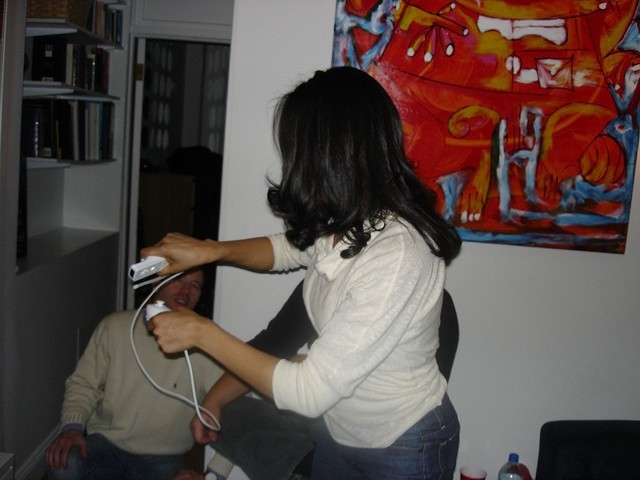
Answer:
[85,53,96,90]
[499,453,524,480]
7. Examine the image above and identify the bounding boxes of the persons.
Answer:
[42,269,224,480]
[138,67,462,480]
[190,277,460,480]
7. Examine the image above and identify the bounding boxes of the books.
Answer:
[91,2,124,43]
[33,36,110,91]
[28,101,114,161]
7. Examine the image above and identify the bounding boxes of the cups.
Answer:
[460,466,486,480]
[24,105,44,157]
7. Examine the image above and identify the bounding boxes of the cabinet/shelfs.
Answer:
[17,0,129,170]
